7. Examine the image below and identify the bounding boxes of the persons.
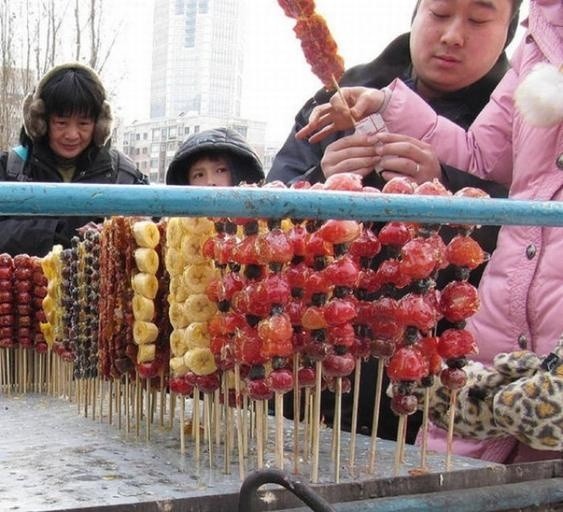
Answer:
[163,127,266,189]
[266,0,520,446]
[293,0,562,461]
[0,62,150,260]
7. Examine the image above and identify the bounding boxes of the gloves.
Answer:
[417,360,510,440]
[493,335,563,451]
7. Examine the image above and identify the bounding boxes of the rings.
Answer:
[415,164,421,176]
